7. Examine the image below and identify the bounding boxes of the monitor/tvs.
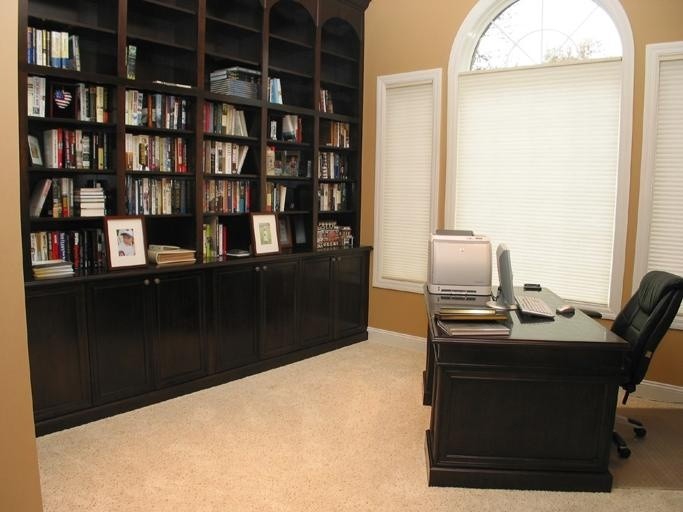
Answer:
[485,241,519,312]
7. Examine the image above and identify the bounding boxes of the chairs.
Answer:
[578,270,683,458]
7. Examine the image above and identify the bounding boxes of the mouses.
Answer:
[556,304,575,315]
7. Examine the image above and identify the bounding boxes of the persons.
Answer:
[117,229,135,257]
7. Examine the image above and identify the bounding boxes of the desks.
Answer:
[421,282,629,493]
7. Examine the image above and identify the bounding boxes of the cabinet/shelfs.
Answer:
[17,1,371,438]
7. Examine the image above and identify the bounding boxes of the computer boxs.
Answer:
[427,234,492,296]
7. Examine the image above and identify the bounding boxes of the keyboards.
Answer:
[515,294,556,319]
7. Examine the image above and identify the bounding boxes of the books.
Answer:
[203,68,261,214]
[317,151,348,180]
[439,304,495,314]
[318,88,333,113]
[319,182,347,212]
[434,311,508,320]
[147,245,198,263]
[204,215,226,257]
[316,221,341,247]
[319,120,349,148]
[267,76,283,104]
[436,320,510,336]
[125,91,192,215]
[126,45,137,79]
[265,113,302,213]
[25,26,111,278]
